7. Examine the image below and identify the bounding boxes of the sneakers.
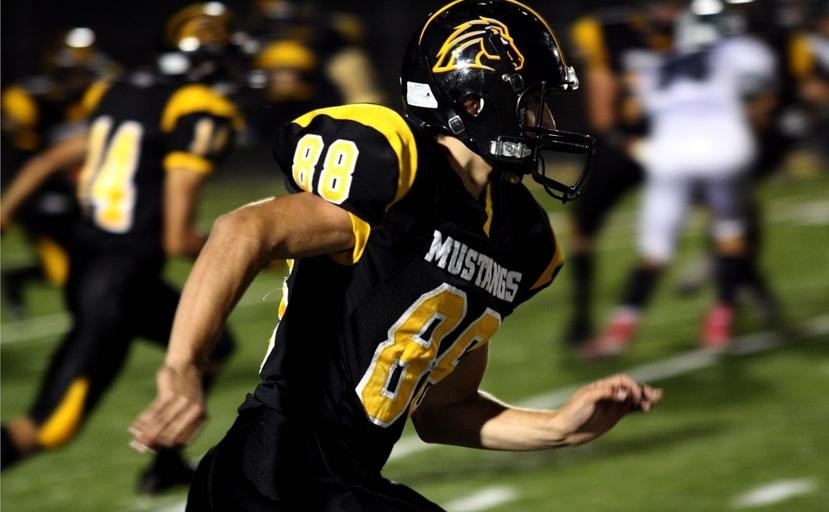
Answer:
[132,459,195,504]
[557,302,783,364]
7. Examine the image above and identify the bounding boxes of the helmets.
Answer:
[398,0,598,206]
[156,1,257,97]
[41,32,98,101]
